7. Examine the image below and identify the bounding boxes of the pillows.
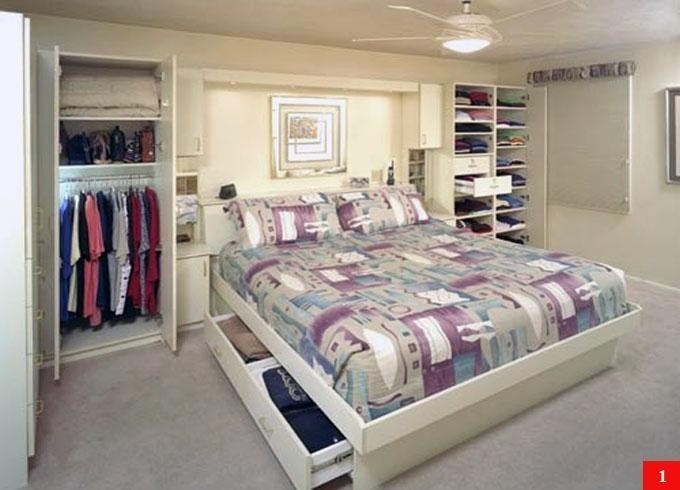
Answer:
[223,185,429,251]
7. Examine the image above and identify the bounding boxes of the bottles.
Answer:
[110,126,125,161]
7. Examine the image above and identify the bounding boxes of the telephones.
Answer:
[386,160,395,185]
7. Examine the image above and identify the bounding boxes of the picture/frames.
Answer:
[665,87,680,183]
[270,96,347,175]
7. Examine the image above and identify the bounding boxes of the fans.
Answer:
[351,0,573,43]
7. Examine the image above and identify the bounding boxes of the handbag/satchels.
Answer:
[220,184,236,198]
[59,121,155,165]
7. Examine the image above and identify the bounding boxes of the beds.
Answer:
[204,186,643,490]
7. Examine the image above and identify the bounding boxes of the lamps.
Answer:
[443,39,490,53]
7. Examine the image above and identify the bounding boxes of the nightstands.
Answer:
[177,238,209,333]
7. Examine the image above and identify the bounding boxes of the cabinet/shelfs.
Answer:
[401,82,443,150]
[426,81,547,248]
[176,68,204,155]
[0,12,45,490]
[401,149,426,195]
[40,45,176,380]
